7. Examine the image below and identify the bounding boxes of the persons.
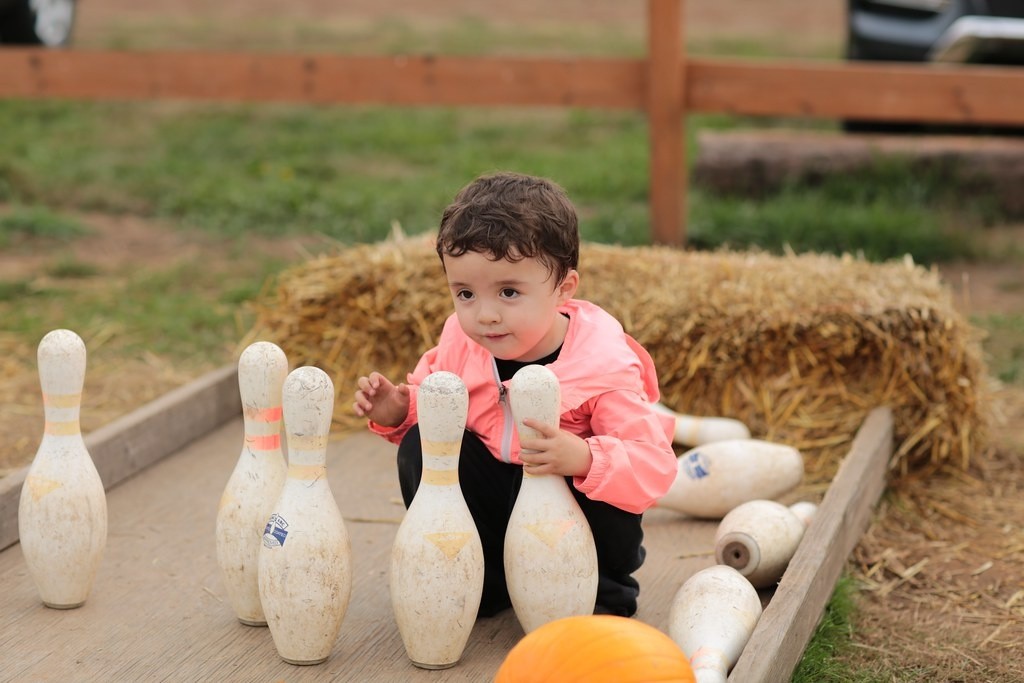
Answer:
[353,174,678,618]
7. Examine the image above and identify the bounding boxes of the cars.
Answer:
[839,0,1024,224]
